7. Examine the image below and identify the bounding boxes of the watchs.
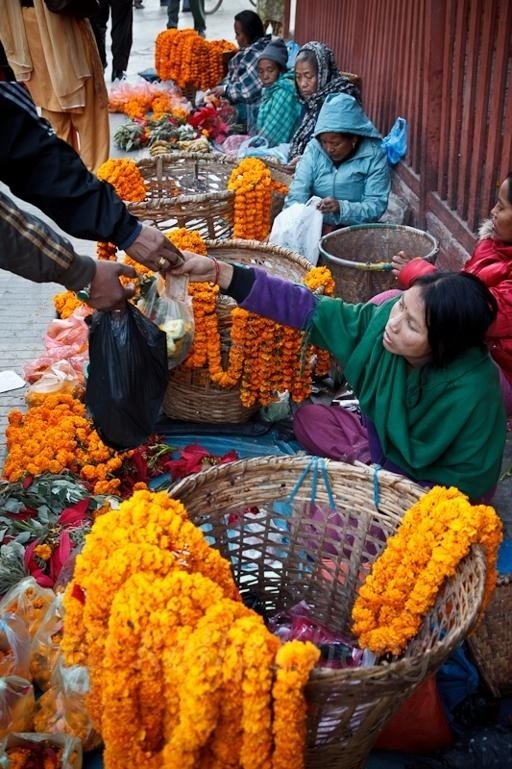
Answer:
[391,173,511,420]
[76,282,93,300]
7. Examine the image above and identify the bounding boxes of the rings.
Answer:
[158,258,167,266]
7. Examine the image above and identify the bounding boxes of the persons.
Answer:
[165,0,206,39]
[205,9,270,135]
[87,2,132,80]
[1,41,187,314]
[254,38,306,148]
[159,1,192,17]
[283,93,391,227]
[167,252,507,724]
[288,41,363,166]
[0,0,111,178]
[133,0,146,9]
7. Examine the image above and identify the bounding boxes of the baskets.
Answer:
[164,457,488,764]
[319,224,439,305]
[116,153,306,237]
[164,237,317,424]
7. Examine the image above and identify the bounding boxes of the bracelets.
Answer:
[208,256,221,289]
